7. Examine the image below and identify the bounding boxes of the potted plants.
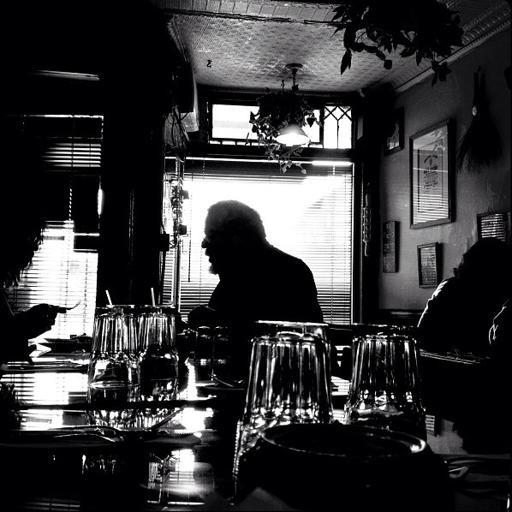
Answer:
[327,0,469,88]
[248,82,319,176]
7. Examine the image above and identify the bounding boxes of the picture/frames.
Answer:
[417,242,441,288]
[381,105,406,156]
[476,208,509,246]
[409,117,457,230]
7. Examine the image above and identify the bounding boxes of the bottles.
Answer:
[193,326,234,366]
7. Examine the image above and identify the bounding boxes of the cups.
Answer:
[243,331,426,432]
[91,306,176,368]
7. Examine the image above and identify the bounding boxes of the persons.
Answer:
[411,237,511,454]
[0,192,68,365]
[187,198,325,374]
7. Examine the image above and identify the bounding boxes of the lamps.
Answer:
[276,62,311,147]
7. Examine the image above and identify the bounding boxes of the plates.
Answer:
[261,424,426,460]
[38,337,86,352]
